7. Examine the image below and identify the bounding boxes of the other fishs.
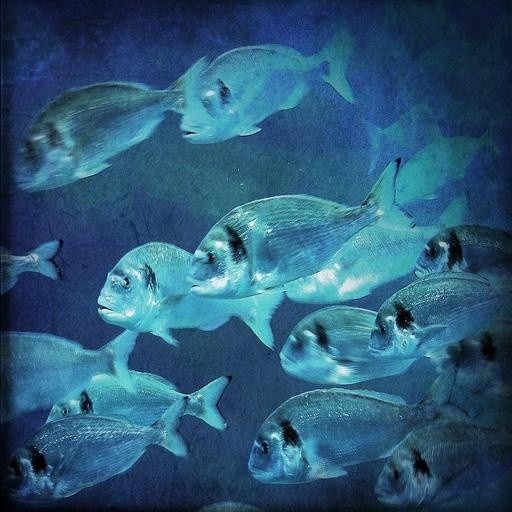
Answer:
[0,193,512,512]
[179,17,357,146]
